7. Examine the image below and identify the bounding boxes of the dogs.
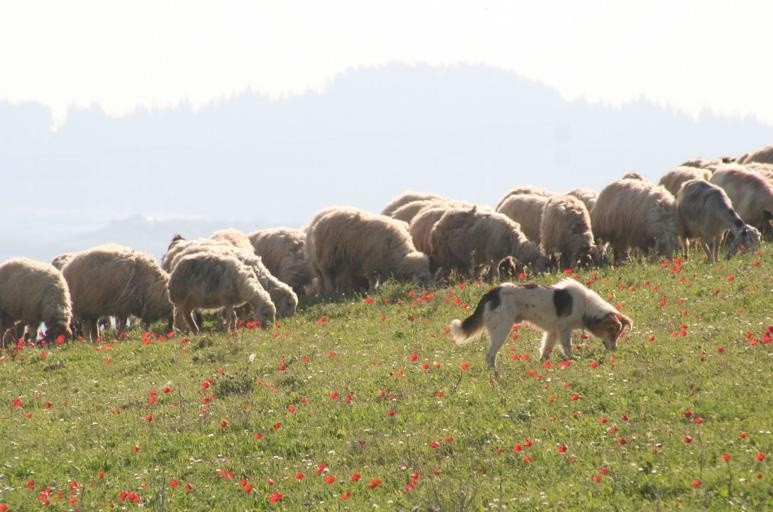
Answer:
[449,277,634,370]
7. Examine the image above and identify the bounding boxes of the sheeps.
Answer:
[0,146,773,348]
